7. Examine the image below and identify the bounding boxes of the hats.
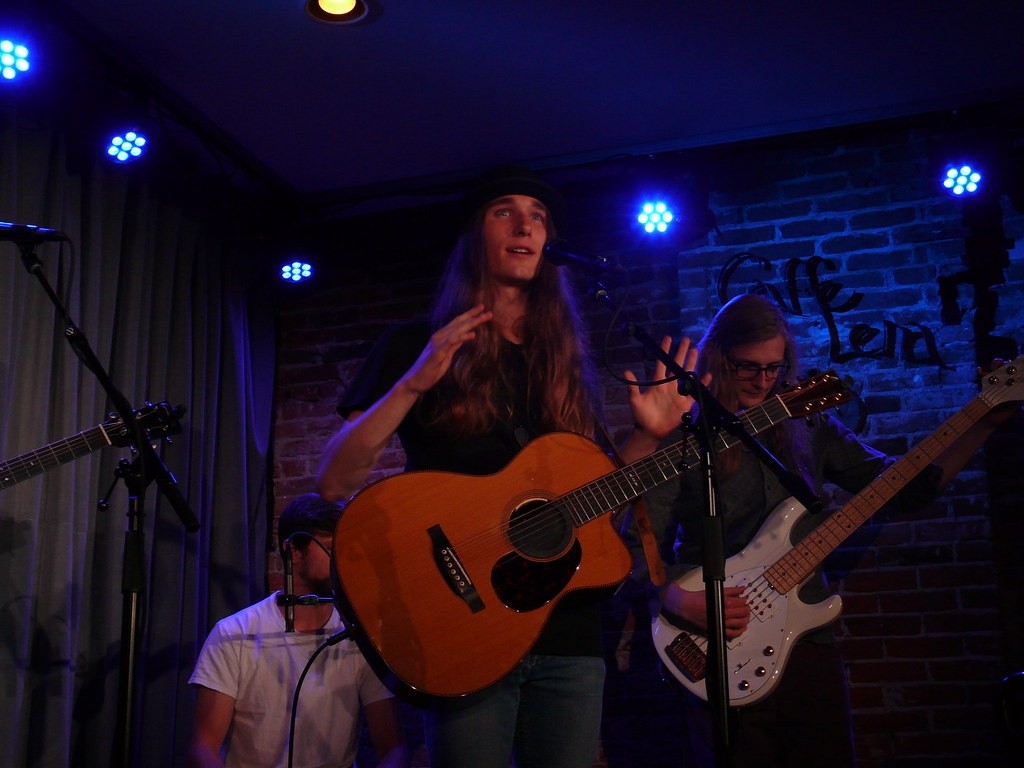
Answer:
[473,163,549,206]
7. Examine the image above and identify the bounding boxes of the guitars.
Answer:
[327,367,864,718]
[647,348,1024,714]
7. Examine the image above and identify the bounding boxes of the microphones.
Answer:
[284,545,295,633]
[0,222,70,242]
[543,238,629,275]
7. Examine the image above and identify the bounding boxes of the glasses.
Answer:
[726,354,784,379]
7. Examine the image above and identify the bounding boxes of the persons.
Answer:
[317,175,712,768]
[621,294,1018,768]
[187,493,408,768]
